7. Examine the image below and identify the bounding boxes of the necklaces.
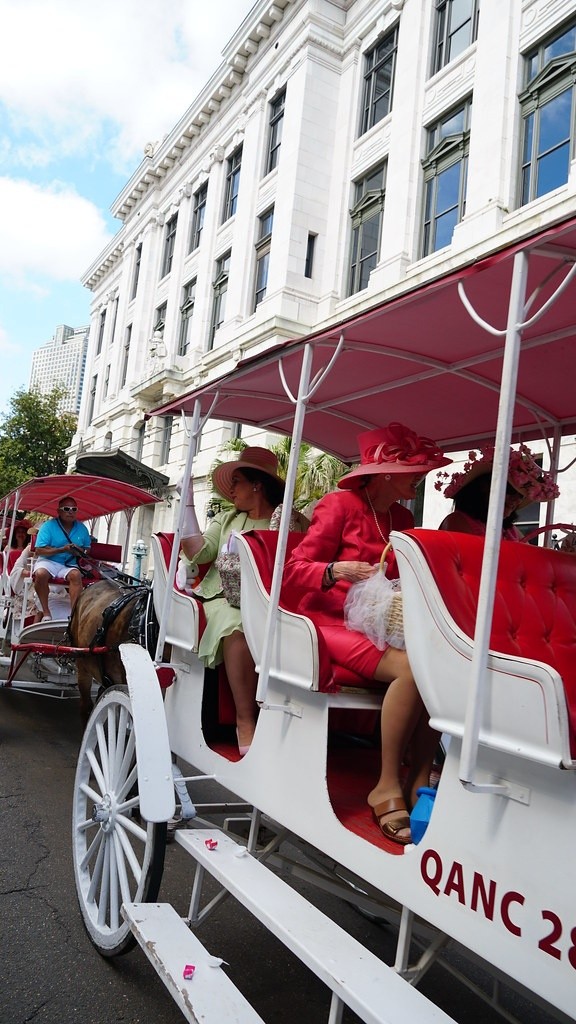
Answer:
[365,487,392,552]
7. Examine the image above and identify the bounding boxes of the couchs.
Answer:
[234,531,387,698]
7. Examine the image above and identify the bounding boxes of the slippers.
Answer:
[374,797,413,845]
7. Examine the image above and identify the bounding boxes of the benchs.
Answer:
[152,533,208,654]
[31,535,122,586]
[388,528,576,771]
[0,550,23,597]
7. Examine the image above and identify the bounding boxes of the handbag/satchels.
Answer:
[215,535,242,610]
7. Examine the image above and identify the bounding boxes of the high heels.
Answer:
[237,724,252,756]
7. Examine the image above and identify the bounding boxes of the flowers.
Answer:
[434,439,561,501]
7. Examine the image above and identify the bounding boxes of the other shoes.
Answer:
[42,615,52,622]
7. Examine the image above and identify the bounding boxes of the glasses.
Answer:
[60,506,78,513]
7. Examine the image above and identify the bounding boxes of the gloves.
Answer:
[176,475,201,540]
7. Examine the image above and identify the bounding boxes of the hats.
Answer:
[212,447,284,504]
[337,423,454,490]
[443,440,559,510]
[5,520,33,538]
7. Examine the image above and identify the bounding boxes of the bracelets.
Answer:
[186,504,195,507]
[327,562,339,583]
[83,548,87,554]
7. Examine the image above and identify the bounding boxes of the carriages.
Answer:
[0,475,172,713]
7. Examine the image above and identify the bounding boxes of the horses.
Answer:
[65,577,158,769]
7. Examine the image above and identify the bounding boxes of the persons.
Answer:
[175,446,311,754]
[434,439,561,545]
[10,520,71,623]
[285,422,453,844]
[34,497,92,622]
[3,518,33,551]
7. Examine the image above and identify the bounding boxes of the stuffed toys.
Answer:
[176,559,201,595]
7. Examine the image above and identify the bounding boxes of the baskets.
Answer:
[347,542,406,652]
